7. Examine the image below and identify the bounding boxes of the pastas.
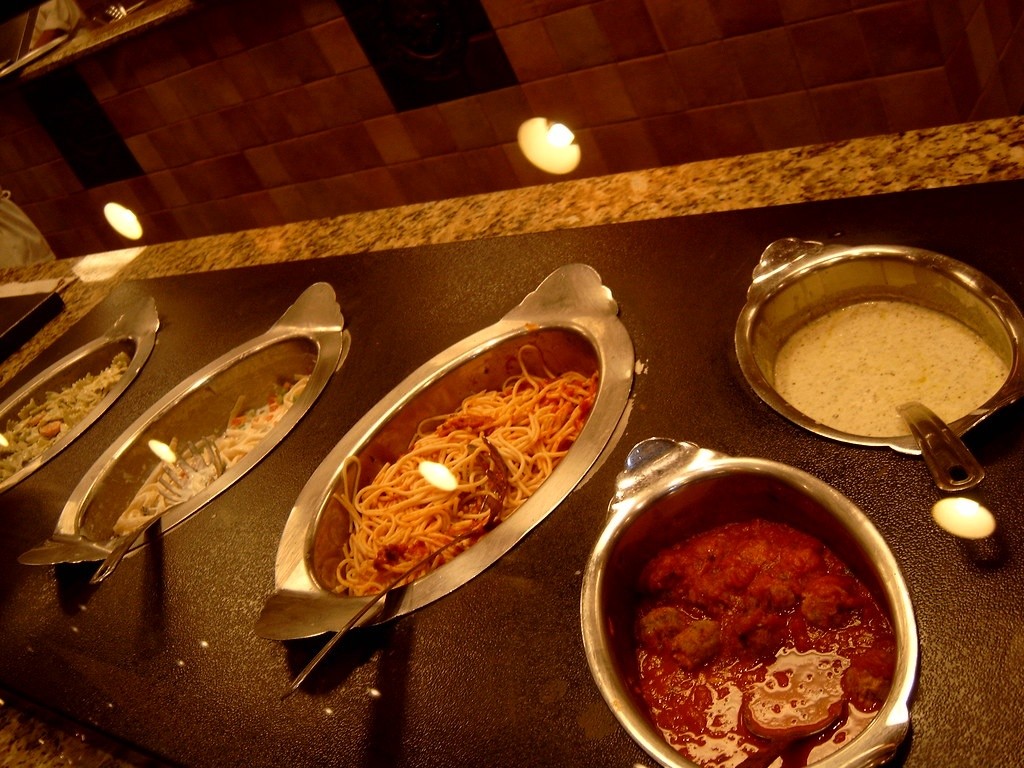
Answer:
[332,345,595,595]
